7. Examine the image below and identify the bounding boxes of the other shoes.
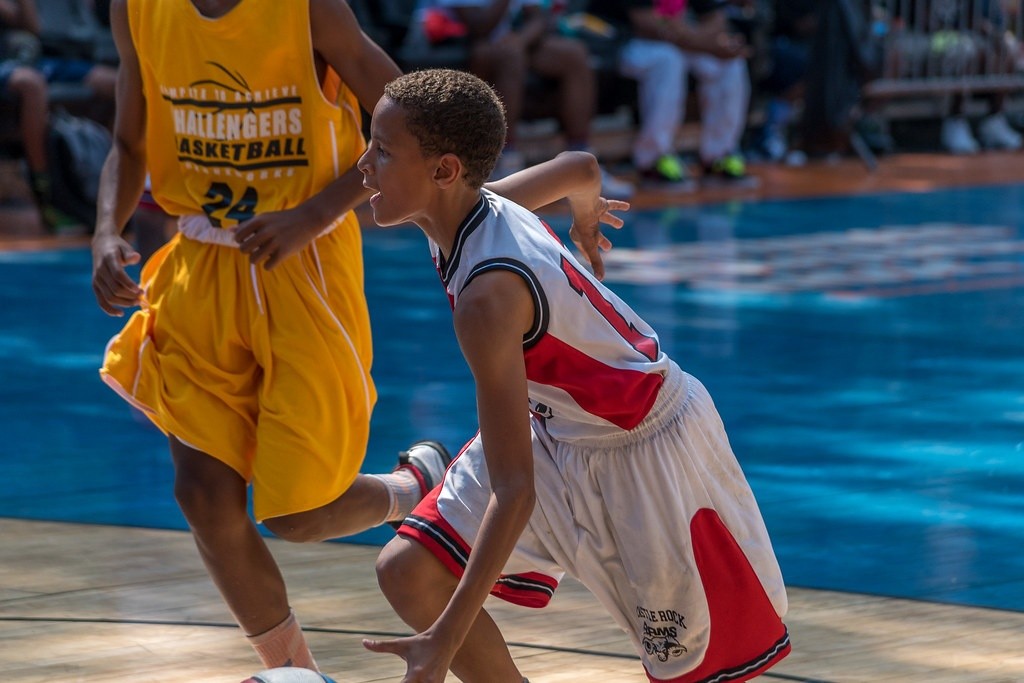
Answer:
[940,115,979,153]
[386,441,451,531]
[643,155,683,180]
[980,115,1023,149]
[600,164,636,200]
[706,155,744,177]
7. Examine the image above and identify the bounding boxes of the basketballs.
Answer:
[237,665,338,683]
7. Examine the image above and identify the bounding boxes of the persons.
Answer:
[359,66,796,683]
[82,0,451,683]
[349,2,1023,196]
[0,0,119,240]
